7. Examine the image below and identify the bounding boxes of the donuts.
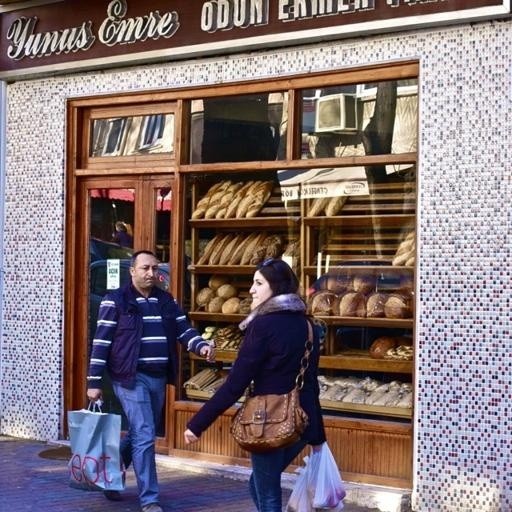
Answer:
[201,326,216,340]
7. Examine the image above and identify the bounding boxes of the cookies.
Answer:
[211,327,245,349]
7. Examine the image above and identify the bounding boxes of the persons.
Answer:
[86,249,218,511]
[111,220,133,248]
[181,256,327,511]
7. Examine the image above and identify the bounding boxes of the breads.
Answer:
[317,375,412,409]
[195,231,282,265]
[191,180,274,218]
[284,240,300,265]
[196,277,253,313]
[305,274,414,319]
[392,229,415,266]
[369,336,414,360]
[307,197,349,217]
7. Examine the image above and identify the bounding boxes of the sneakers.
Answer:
[140,503,163,512]
[103,490,123,501]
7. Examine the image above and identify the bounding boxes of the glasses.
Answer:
[261,257,280,273]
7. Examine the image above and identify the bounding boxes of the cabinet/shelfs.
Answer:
[184,180,416,422]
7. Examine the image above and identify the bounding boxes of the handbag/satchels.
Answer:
[230,385,310,453]
[67,398,126,493]
